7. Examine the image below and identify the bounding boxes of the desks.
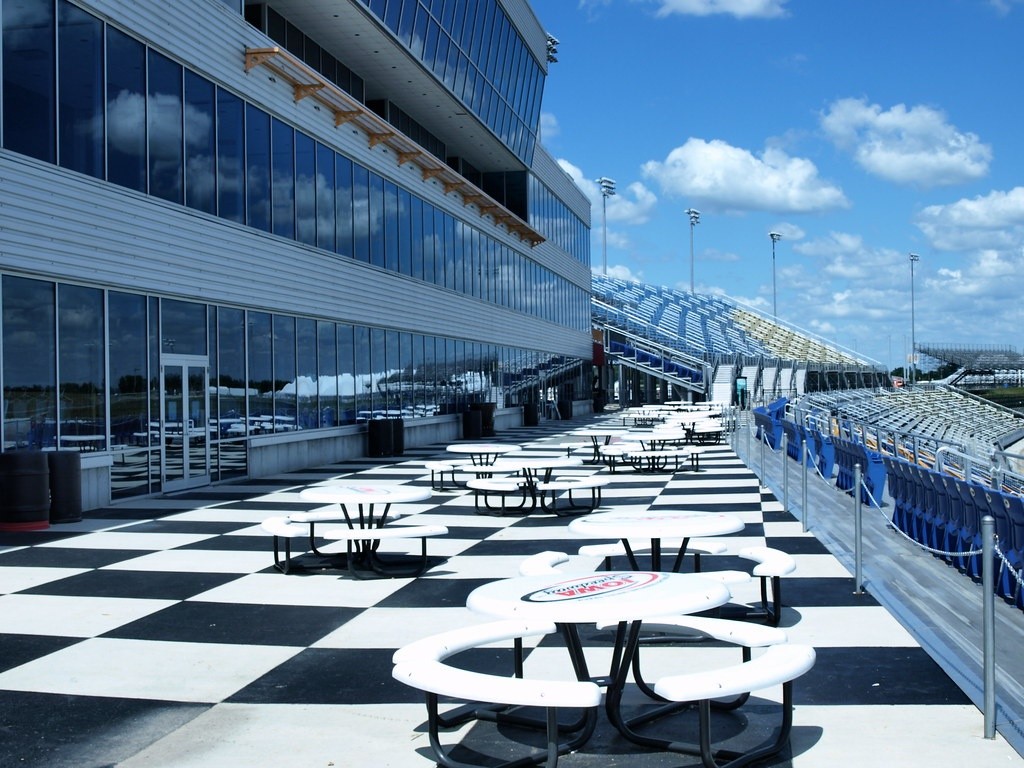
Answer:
[466,566,739,748]
[629,407,660,425]
[443,436,521,480]
[627,430,687,468]
[302,481,433,569]
[504,452,582,512]
[562,508,747,573]
[664,400,691,412]
[668,415,708,443]
[569,426,629,461]
[693,401,722,412]
[670,411,710,423]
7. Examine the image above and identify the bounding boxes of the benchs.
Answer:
[263,401,816,768]
